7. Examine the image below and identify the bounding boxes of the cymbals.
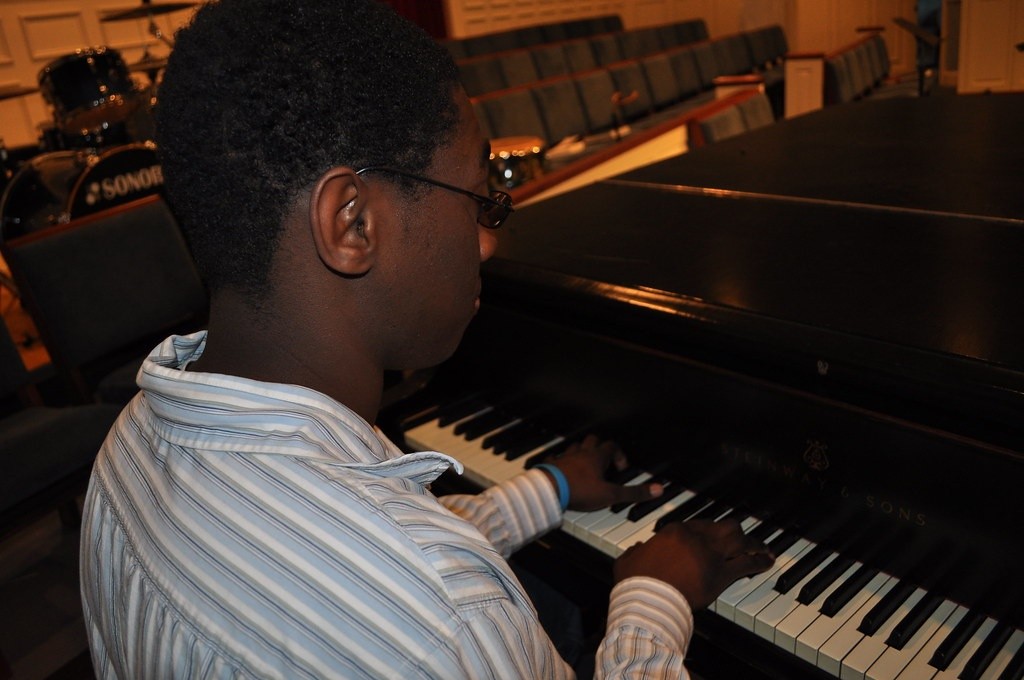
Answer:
[124,52,174,69]
[0,83,45,102]
[99,3,198,20]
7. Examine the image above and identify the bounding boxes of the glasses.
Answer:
[356,167,515,229]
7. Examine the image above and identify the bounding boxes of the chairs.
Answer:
[0,312,122,537]
[0,193,211,406]
[0,147,97,242]
[66,142,176,222]
[437,12,898,210]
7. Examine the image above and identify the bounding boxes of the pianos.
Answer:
[375,92,1023,680]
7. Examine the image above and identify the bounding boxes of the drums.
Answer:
[1,143,164,242]
[36,43,141,117]
[483,133,544,190]
[45,99,152,152]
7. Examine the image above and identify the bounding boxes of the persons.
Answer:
[78,0,780,680]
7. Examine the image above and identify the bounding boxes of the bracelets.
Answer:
[530,463,569,514]
